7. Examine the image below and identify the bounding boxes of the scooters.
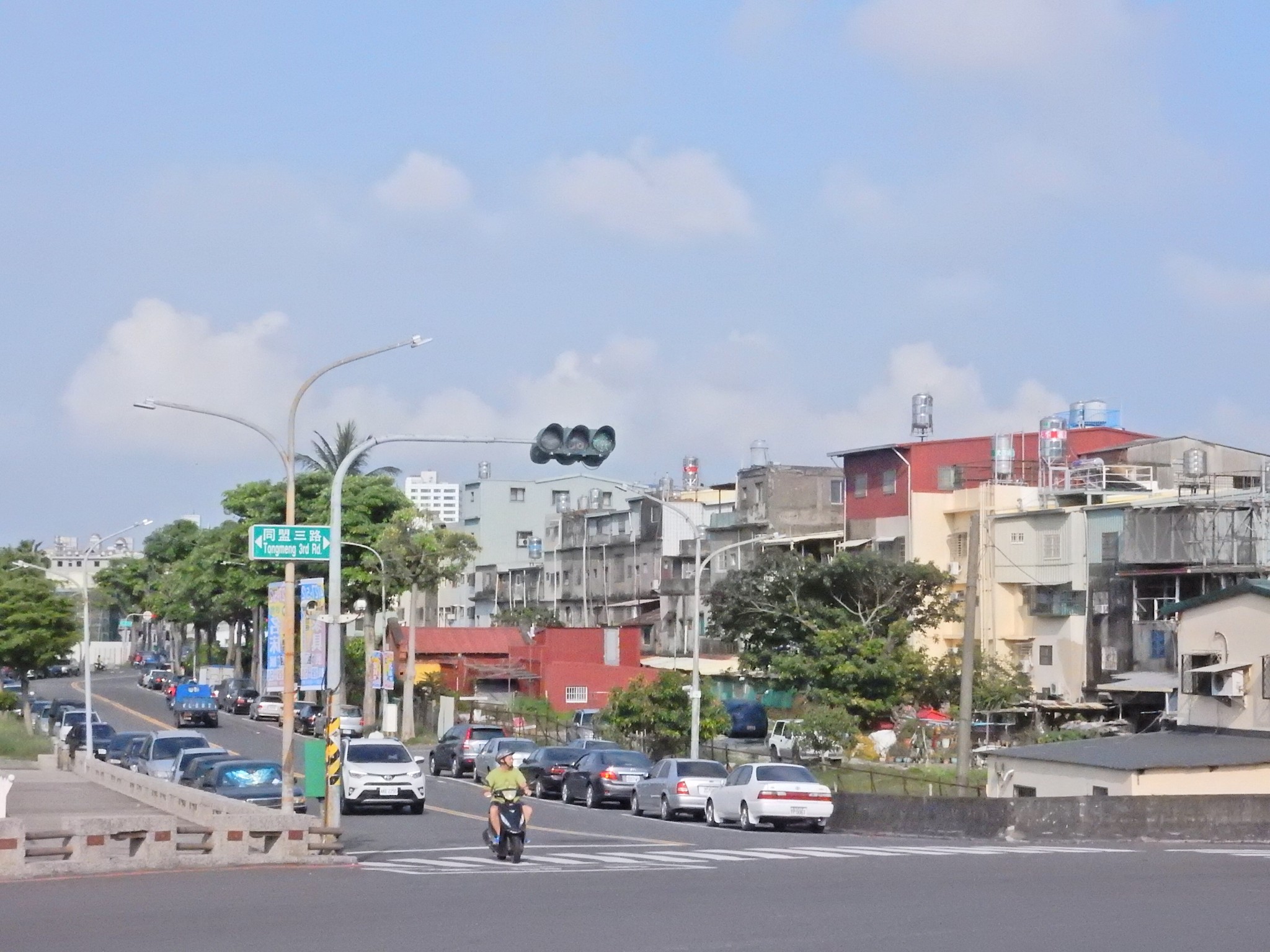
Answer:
[480,778,527,864]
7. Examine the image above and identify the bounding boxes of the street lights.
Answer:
[617,480,781,761]
[127,332,438,817]
[12,515,155,762]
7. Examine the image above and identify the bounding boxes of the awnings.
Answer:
[1098,670,1181,696]
[1186,659,1254,676]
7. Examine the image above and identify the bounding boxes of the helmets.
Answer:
[495,748,515,761]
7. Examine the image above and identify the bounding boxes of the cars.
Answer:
[103,731,149,768]
[704,760,835,833]
[295,703,321,737]
[250,694,284,722]
[719,698,766,737]
[65,722,118,759]
[629,758,729,821]
[120,737,152,772]
[518,746,571,798]
[570,739,621,755]
[133,648,259,715]
[472,736,538,788]
[561,748,651,809]
[179,754,246,795]
[279,701,315,728]
[169,747,232,795]
[195,758,306,816]
[0,658,101,741]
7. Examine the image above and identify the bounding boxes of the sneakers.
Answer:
[524,837,530,843]
[492,835,500,845]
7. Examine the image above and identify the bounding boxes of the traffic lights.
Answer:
[537,423,617,459]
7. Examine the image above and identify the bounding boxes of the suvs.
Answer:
[137,729,211,783]
[571,708,603,740]
[314,703,363,738]
[427,724,509,777]
[339,736,425,812]
[769,719,845,767]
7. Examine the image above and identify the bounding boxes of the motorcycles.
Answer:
[94,661,108,671]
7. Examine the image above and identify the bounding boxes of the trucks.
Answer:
[173,683,220,727]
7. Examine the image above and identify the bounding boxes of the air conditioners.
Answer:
[946,561,960,574]
[1209,669,1245,698]
[1014,660,1032,674]
[1048,681,1064,697]
[649,578,661,592]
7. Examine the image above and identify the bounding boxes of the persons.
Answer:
[484,747,533,845]
